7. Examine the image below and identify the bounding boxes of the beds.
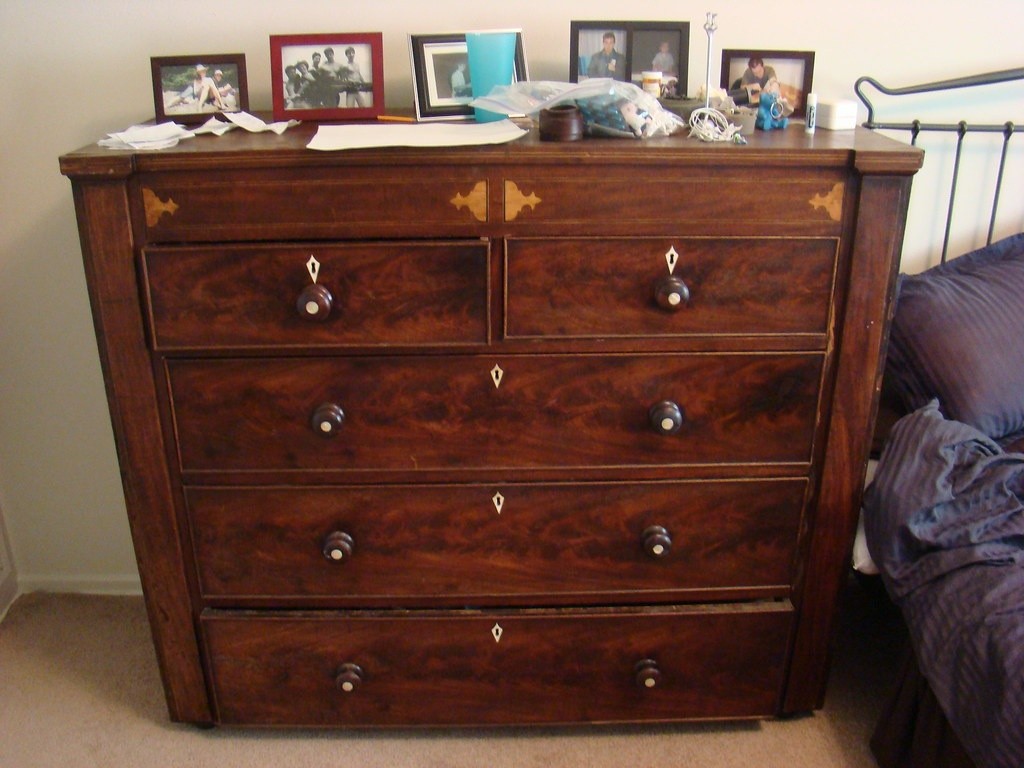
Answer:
[853,67,1024,768]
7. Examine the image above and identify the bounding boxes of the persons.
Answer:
[167,65,237,111]
[653,41,673,75]
[283,46,368,107]
[732,57,780,94]
[449,56,472,97]
[587,30,626,83]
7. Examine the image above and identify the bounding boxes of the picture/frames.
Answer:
[151,53,249,124]
[569,20,690,101]
[407,29,529,122]
[270,32,385,122]
[720,48,815,118]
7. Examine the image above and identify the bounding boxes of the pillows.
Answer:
[883,233,1023,442]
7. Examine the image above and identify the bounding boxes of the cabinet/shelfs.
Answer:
[56,127,924,730]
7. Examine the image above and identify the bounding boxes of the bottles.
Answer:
[642,71,663,100]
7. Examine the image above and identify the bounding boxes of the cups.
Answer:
[465,31,517,123]
